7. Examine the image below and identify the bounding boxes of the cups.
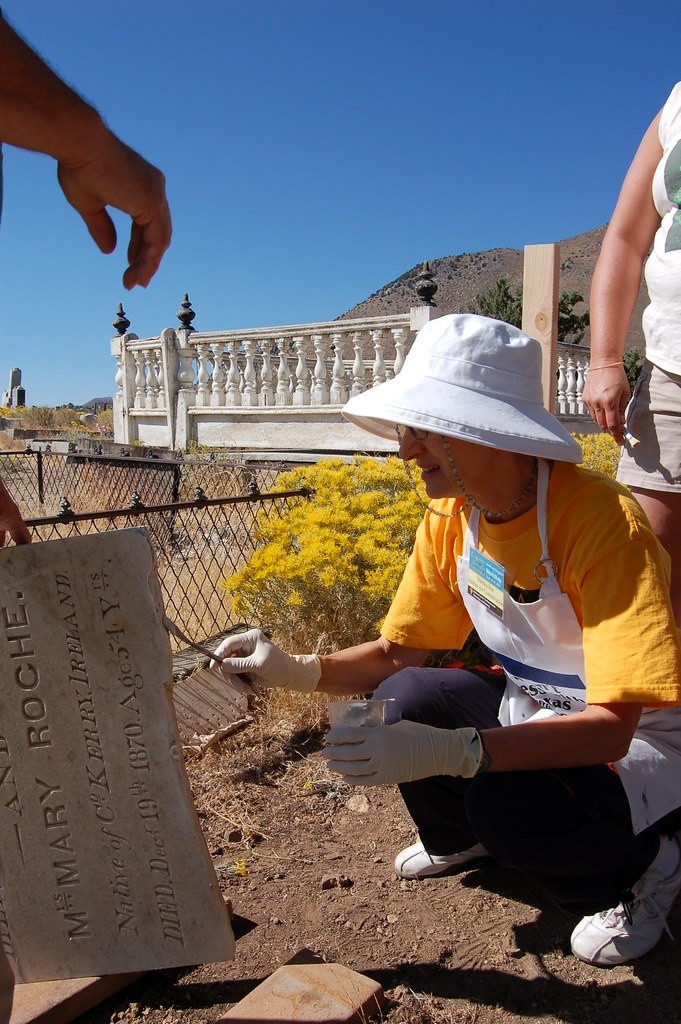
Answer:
[328,701,387,774]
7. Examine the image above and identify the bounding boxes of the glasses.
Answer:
[393,424,429,440]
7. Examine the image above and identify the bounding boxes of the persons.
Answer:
[0,8,172,290]
[206,312,681,967]
[578,81,681,653]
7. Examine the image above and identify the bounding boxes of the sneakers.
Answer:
[569,834,681,965]
[394,840,490,879]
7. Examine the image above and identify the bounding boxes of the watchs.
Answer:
[456,728,491,774]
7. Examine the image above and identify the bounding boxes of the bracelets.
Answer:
[585,362,624,371]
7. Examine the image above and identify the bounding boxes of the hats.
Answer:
[341,313,583,464]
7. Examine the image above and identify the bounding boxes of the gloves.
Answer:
[322,720,483,786]
[209,629,321,694]
